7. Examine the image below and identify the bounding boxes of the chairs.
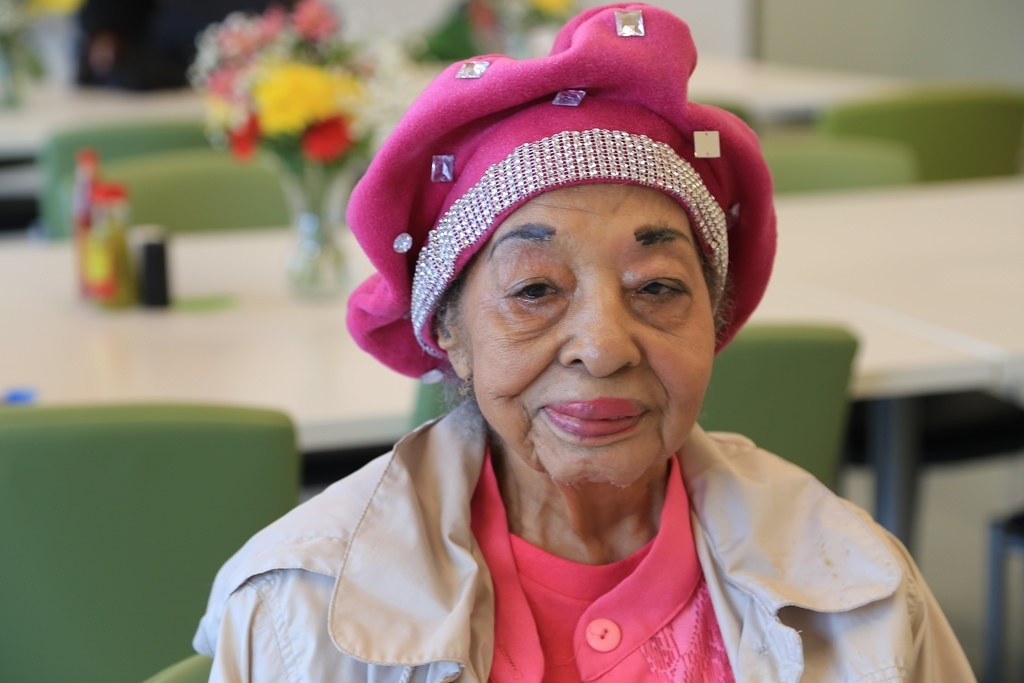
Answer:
[0,30,1024,683]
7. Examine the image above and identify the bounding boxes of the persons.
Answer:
[204,2,979,681]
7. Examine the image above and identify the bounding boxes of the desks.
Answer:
[0,39,890,165]
[0,171,1024,542]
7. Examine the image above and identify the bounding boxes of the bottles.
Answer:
[80,183,144,308]
[73,148,105,257]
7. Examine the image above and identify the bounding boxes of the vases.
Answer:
[266,165,372,316]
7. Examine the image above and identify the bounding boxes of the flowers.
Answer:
[188,4,396,203]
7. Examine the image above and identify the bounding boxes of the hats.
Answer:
[344,3,777,378]
